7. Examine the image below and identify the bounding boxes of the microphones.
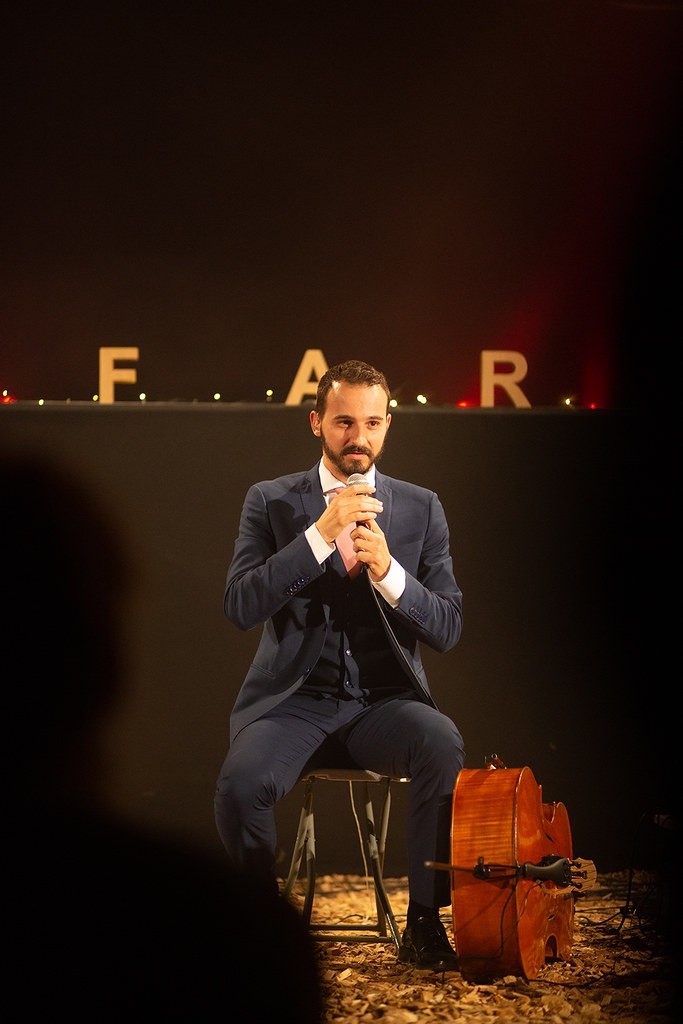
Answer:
[348,474,371,567]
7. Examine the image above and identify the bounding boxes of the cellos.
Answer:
[424,766,598,986]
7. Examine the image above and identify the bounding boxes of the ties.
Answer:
[335,487,363,581]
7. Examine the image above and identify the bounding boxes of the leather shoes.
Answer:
[399,916,459,973]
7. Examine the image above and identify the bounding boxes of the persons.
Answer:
[212,359,464,972]
[0,447,330,1024]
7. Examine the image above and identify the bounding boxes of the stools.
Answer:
[279,763,411,949]
